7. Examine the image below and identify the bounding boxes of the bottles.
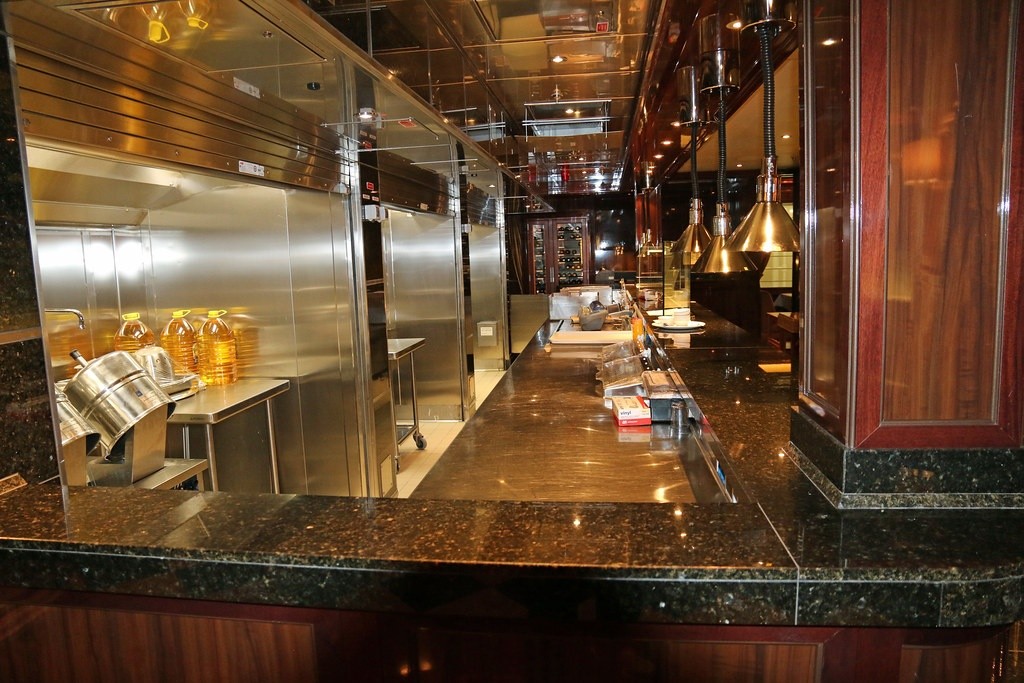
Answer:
[113,312,155,353]
[196,309,238,385]
[160,309,196,377]
[534,226,582,293]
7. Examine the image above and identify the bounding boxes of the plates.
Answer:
[652,321,706,329]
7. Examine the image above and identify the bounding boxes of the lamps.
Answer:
[669,123,712,254]
[718,23,800,252]
[688,88,758,273]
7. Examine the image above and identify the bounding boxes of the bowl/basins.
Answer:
[659,316,673,326]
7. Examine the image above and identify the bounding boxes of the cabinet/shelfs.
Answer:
[526,213,594,294]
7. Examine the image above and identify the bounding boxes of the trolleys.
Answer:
[388,337,429,475]
[167,379,290,494]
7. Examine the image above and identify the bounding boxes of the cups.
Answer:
[671,404,689,429]
[673,309,689,325]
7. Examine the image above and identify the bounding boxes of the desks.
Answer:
[166,377,291,493]
[387,337,426,443]
